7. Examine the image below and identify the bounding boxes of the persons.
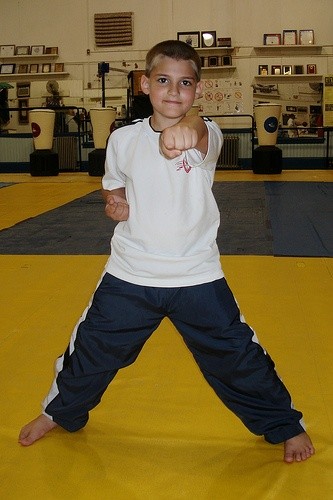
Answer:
[18,40,315,463]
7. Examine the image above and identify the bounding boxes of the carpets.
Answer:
[0,181,333,258]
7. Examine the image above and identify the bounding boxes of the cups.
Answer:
[254,103,282,146]
[89,108,116,149]
[186,105,199,117]
[28,109,56,149]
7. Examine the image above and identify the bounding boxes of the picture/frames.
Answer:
[0,44,58,56]
[258,63,316,75]
[0,63,64,74]
[176,31,232,67]
[283,29,297,45]
[263,33,281,45]
[299,29,314,45]
